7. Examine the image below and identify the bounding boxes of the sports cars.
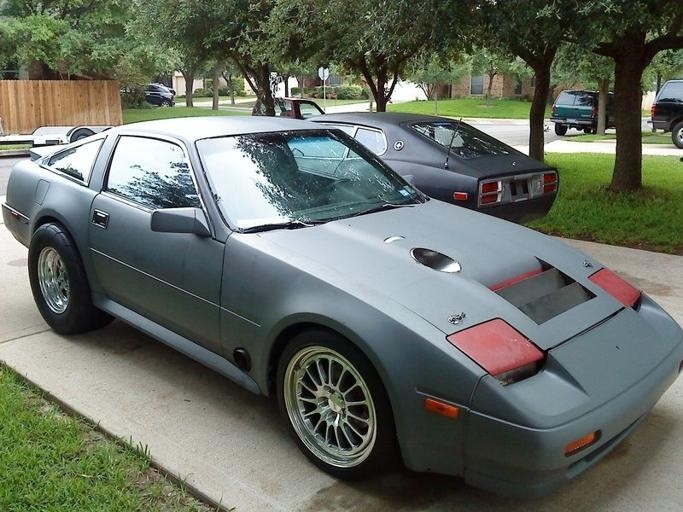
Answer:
[1,111,683,502]
[284,112,560,225]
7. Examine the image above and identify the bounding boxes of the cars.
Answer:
[121,83,176,107]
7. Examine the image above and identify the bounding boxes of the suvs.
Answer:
[646,79,683,149]
[550,90,616,136]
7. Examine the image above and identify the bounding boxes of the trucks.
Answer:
[0,79,124,146]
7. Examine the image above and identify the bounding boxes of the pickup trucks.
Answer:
[251,96,326,121]
